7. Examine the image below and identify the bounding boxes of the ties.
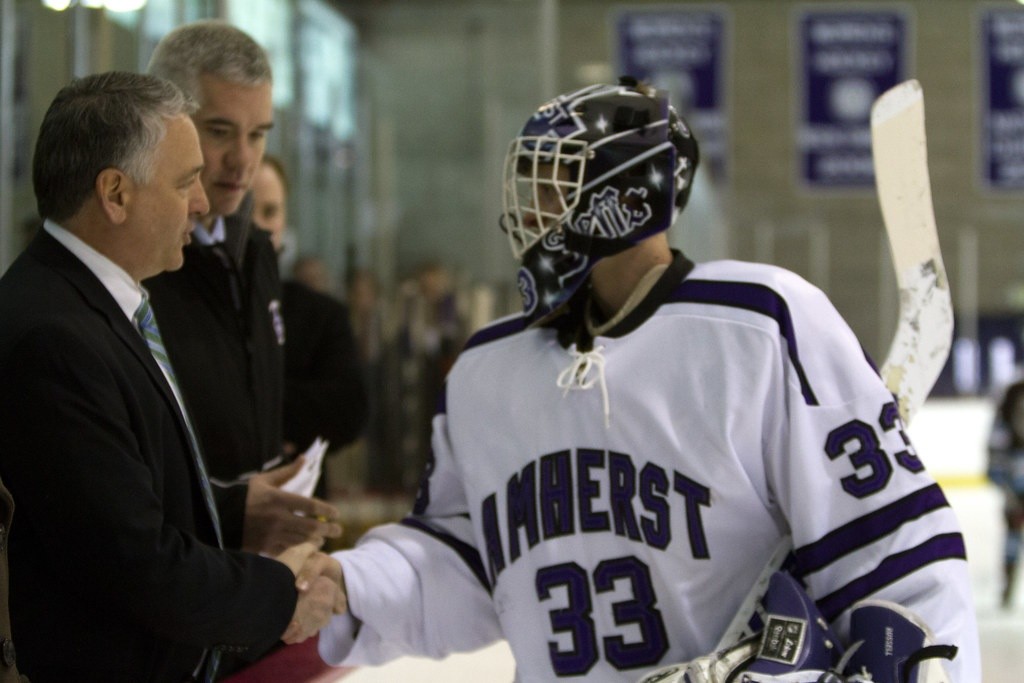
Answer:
[134,300,226,551]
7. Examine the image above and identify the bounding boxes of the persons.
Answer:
[297,76,1024,683]
[0,21,372,683]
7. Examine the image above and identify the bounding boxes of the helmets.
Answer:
[501,74,700,324]
